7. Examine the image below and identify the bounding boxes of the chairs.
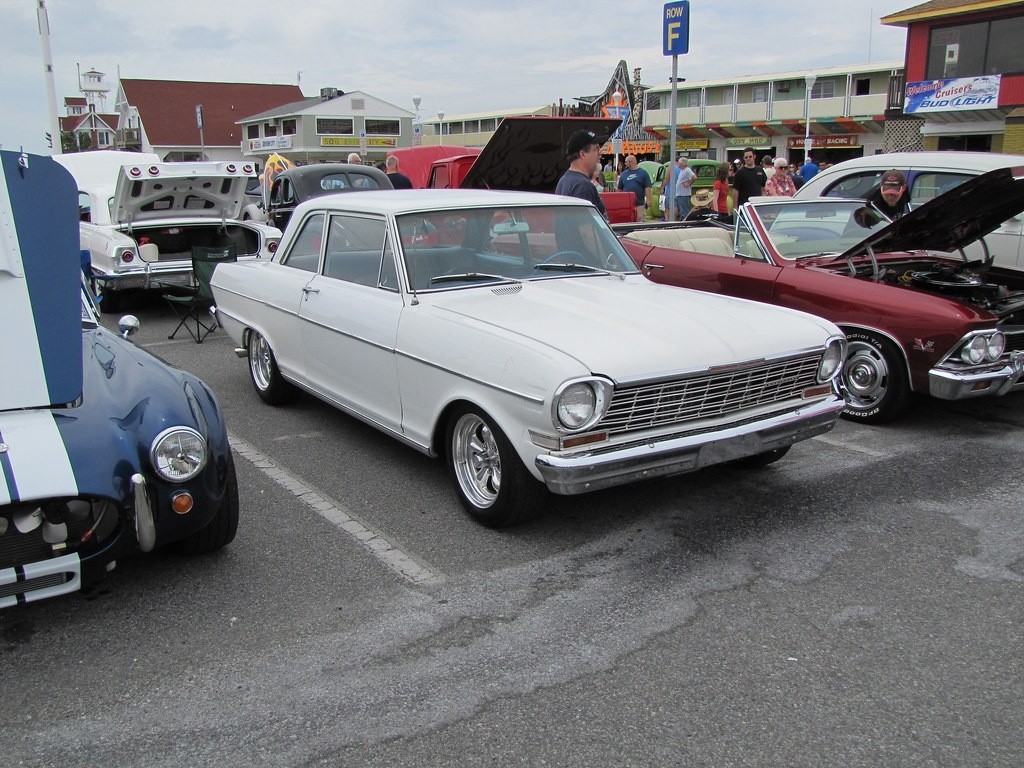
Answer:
[157,242,238,343]
[288,246,480,289]
[80,249,121,304]
[678,238,734,257]
[747,236,789,257]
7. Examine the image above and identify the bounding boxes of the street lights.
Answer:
[412,94,422,146]
[437,111,445,146]
[611,90,623,173]
[804,69,817,166]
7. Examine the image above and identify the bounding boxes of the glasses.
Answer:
[585,147,601,156]
[744,156,753,159]
[777,166,789,171]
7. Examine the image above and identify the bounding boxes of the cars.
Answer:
[240,163,438,256]
[385,114,640,261]
[207,187,851,531]
[51,150,283,315]
[766,150,1024,272]
[638,157,723,222]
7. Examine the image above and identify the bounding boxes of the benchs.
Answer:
[625,226,733,257]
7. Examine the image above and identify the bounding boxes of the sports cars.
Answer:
[0,149,241,613]
[606,165,1023,427]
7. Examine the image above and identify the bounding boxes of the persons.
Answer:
[660,156,681,221]
[368,156,413,189]
[713,163,729,216]
[675,157,696,220]
[341,153,364,187]
[554,130,611,264]
[616,155,652,222]
[591,159,625,192]
[728,155,833,197]
[843,169,911,237]
[733,148,767,210]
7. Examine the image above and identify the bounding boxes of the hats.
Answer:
[567,129,609,155]
[690,188,715,207]
[854,207,873,230]
[880,168,906,195]
